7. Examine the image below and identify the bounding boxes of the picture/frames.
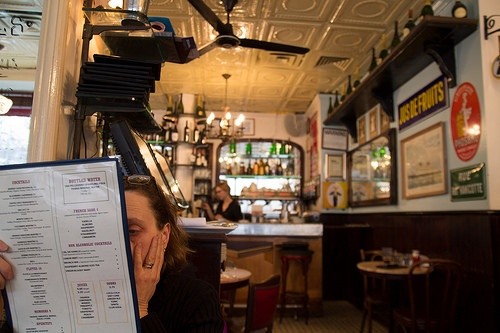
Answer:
[346,129,397,208]
[398,121,448,199]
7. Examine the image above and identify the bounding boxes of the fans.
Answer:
[189,0,310,55]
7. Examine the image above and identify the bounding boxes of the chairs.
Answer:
[360,249,468,333]
[224,274,281,333]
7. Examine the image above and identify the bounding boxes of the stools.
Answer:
[278,248,313,326]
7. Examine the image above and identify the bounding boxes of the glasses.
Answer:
[122,174,153,188]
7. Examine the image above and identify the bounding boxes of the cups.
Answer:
[382,247,411,264]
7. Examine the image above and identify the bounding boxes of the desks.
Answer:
[356,261,432,333]
[220,267,253,333]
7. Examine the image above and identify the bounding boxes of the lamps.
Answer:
[205,73,246,141]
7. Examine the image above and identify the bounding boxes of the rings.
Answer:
[143,261,154,268]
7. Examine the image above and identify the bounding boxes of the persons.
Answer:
[202,182,243,222]
[0,175,224,333]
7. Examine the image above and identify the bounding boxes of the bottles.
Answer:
[225,156,294,176]
[412,250,420,270]
[328,0,467,115]
[372,145,391,178]
[184,120,189,142]
[229,139,291,155]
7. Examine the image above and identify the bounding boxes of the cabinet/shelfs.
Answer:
[72,0,163,159]
[149,113,303,217]
